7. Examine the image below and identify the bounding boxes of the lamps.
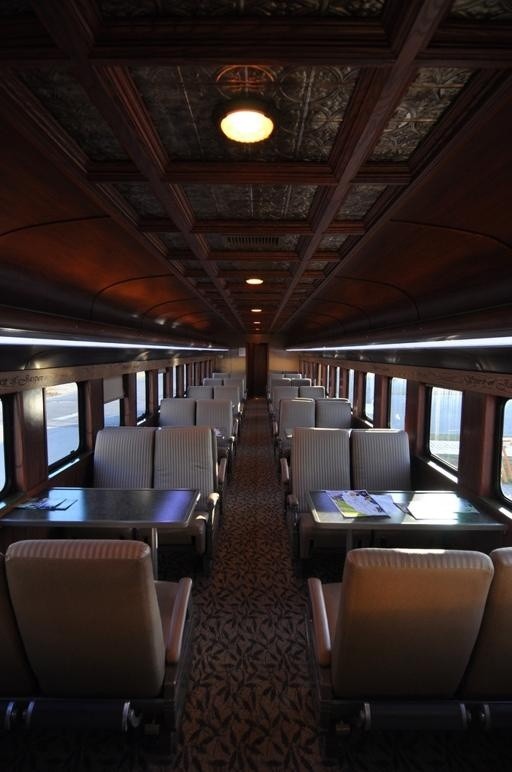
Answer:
[218,98,275,146]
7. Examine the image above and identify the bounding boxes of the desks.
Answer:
[0,487,201,579]
[297,490,507,570]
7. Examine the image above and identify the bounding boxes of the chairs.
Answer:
[135,427,220,576]
[268,370,353,452]
[469,548,512,735]
[307,547,495,736]
[6,540,193,755]
[92,429,156,489]
[0,553,35,698]
[280,428,372,559]
[163,426,227,493]
[351,429,431,539]
[158,372,246,469]
